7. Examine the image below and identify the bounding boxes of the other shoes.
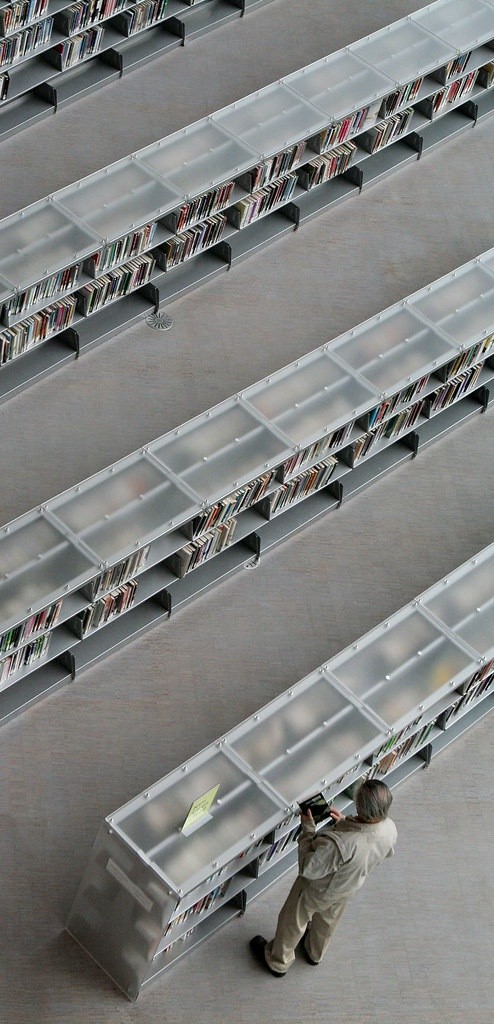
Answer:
[250,935,287,977]
[300,934,320,965]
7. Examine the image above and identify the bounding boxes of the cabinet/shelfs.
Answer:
[66,541,493,1004]
[0,246,494,744]
[0,0,494,406]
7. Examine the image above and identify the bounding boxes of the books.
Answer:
[0,600,62,684]
[170,421,355,578]
[345,335,494,466]
[1,53,494,361]
[445,658,494,721]
[153,761,363,960]
[78,546,150,634]
[345,714,436,799]
[0,0,168,101]
[296,792,333,826]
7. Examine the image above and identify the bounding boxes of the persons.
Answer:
[250,779,397,977]
